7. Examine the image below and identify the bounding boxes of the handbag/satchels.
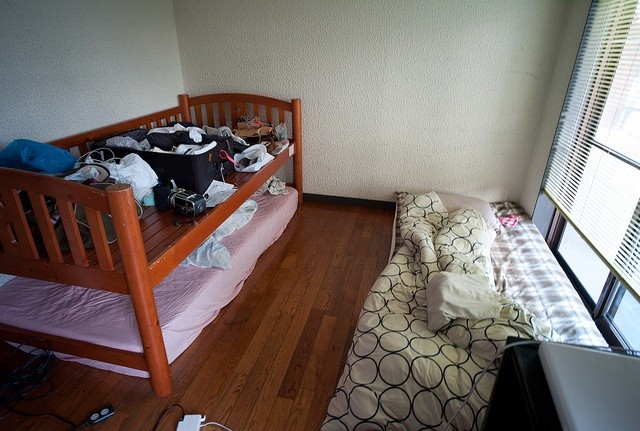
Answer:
[15,164,144,257]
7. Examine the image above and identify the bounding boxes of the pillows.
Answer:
[437,193,499,231]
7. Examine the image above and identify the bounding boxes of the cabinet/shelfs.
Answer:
[480,334,562,430]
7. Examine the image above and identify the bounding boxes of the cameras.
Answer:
[168,187,206,227]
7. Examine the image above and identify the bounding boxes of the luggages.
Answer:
[91,127,236,196]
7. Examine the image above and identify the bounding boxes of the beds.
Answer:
[0,92,304,400]
[321,190,612,430]
